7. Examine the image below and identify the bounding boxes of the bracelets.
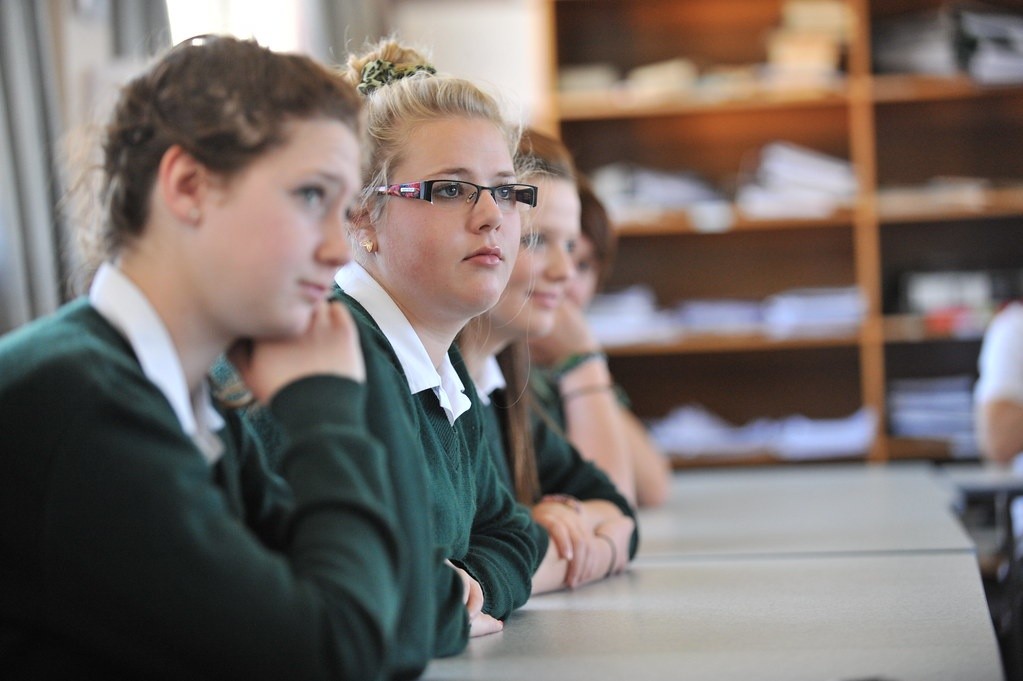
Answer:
[595,532,617,577]
[553,352,607,382]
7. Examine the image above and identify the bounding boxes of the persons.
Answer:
[530,185,675,507]
[0,34,433,681]
[974,301,1022,680]
[459,124,639,597]
[326,43,549,661]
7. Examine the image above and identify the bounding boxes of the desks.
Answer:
[634,460,972,553]
[419,553,1006,680]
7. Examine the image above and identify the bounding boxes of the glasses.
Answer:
[361,180,538,211]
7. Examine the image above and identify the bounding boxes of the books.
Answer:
[892,373,981,452]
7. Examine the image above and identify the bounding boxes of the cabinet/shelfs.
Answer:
[543,0,1023,471]
